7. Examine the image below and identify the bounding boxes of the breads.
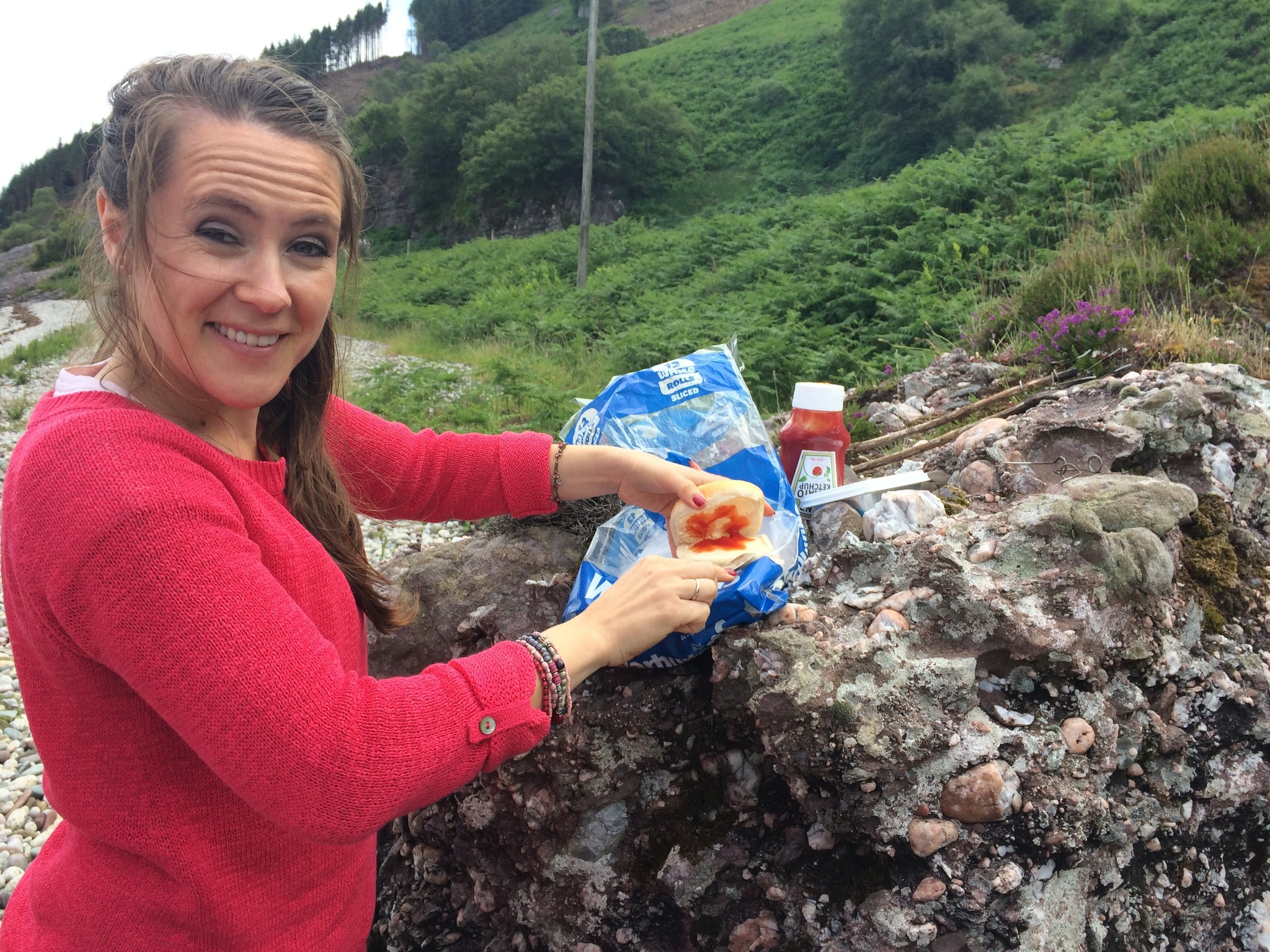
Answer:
[669,481,777,565]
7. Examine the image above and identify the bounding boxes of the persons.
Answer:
[0,55,775,952]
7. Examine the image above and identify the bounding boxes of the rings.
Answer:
[690,578,700,600]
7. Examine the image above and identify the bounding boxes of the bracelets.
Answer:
[515,631,573,726]
[551,441,569,506]
